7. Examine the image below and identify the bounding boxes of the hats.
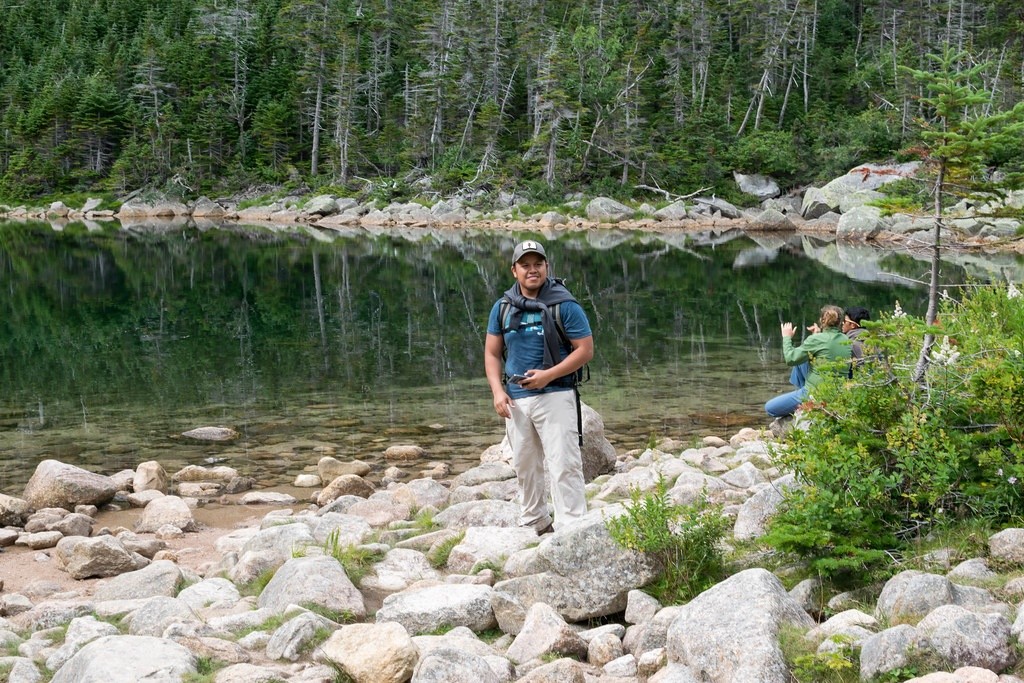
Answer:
[512,240,547,265]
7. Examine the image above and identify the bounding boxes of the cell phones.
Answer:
[509,373,531,385]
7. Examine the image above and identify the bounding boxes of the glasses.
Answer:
[844,318,861,327]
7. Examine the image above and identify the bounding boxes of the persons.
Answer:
[484,239,594,536]
[764,304,883,418]
